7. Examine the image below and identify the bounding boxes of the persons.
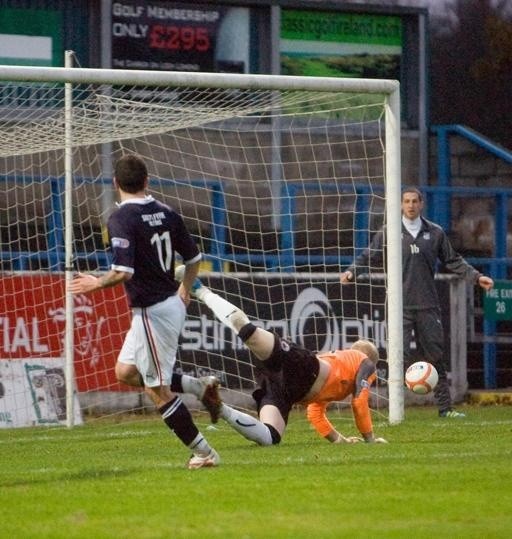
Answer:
[340,186,493,417]
[175,265,386,445]
[68,154,222,469]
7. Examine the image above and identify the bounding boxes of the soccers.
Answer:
[406,362,439,395]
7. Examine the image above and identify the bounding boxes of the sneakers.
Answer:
[198,375,222,424]
[174,264,203,297]
[439,408,466,418]
[185,447,220,469]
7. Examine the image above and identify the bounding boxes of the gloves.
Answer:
[325,428,387,444]
